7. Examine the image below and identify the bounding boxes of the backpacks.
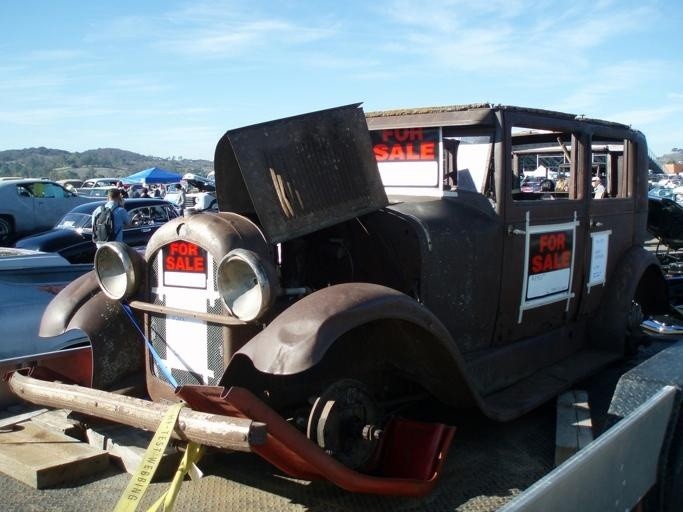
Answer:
[92,205,121,242]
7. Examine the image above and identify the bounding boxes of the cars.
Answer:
[37,101,683,499]
[0,174,220,265]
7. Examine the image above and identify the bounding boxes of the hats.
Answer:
[175,184,181,188]
[592,177,601,181]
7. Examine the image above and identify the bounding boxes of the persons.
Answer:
[540,180,555,192]
[92,188,131,249]
[117,181,166,198]
[174,184,186,216]
[592,177,606,199]
